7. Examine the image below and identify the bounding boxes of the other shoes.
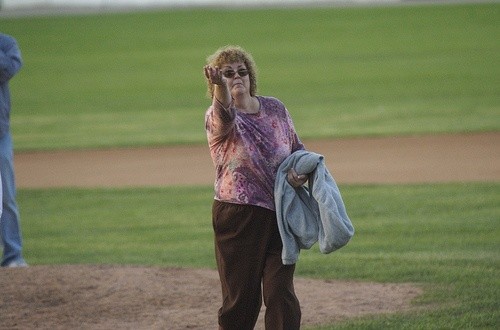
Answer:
[9,259,28,268]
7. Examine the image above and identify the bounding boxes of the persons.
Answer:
[204,45,310,330]
[0,32,28,268]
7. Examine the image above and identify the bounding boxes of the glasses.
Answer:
[223,69,249,78]
